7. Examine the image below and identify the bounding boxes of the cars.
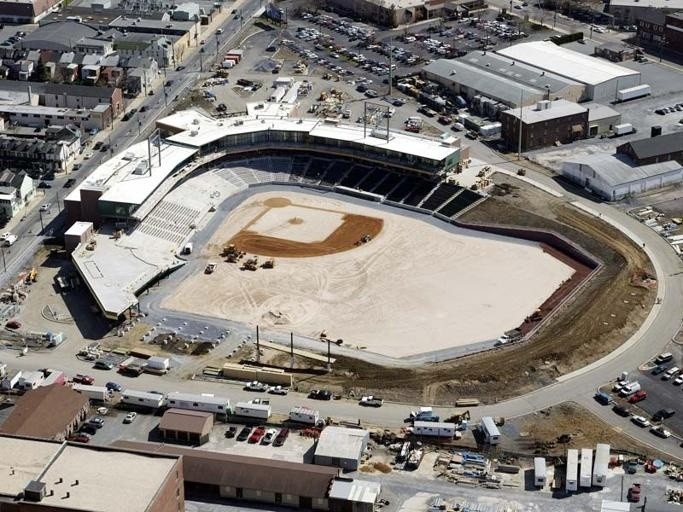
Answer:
[627,459,638,474]
[310,388,332,400]
[40,202,51,212]
[0,232,19,246]
[361,395,384,407]
[653,352,683,385]
[225,423,290,447]
[594,379,676,438]
[72,356,145,392]
[62,141,111,188]
[245,380,290,396]
[66,410,137,443]
[630,486,641,503]
[217,27,225,34]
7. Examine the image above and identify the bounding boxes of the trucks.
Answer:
[498,329,522,345]
